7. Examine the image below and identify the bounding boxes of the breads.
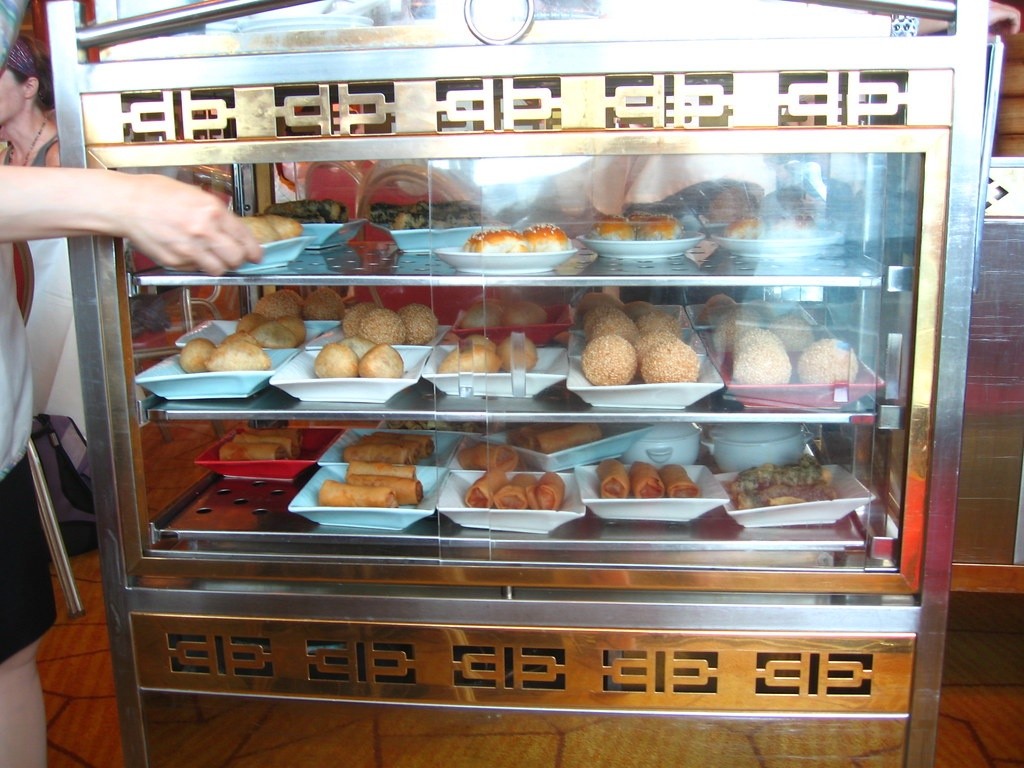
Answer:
[573,290,736,321]
[990,32,1024,158]
[178,299,547,377]
[235,178,827,256]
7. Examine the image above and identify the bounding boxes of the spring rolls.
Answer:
[455,421,602,511]
[218,426,304,460]
[319,432,436,510]
[596,458,701,498]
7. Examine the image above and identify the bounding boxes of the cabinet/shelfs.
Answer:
[138,227,908,557]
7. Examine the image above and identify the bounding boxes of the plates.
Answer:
[713,464,876,528]
[239,14,375,32]
[169,21,238,37]
[134,304,885,408]
[152,219,841,274]
[573,465,730,522]
[193,423,655,534]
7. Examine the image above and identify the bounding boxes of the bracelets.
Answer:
[889,13,919,37]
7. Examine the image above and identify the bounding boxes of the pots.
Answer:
[700,422,814,472]
[615,422,703,465]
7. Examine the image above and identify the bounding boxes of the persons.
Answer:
[1,154,262,768]
[0,36,108,561]
[838,0,1024,38]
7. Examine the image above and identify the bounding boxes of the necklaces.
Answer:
[9,118,47,167]
